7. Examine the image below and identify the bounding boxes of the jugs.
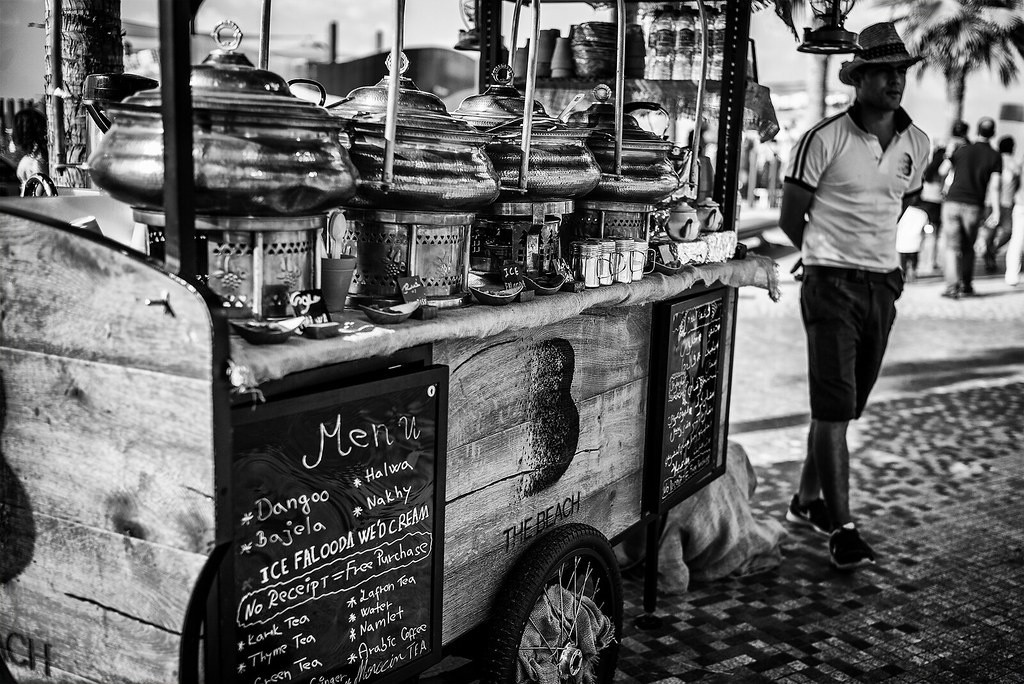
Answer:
[667,196,723,240]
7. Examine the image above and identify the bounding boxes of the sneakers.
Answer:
[786,494,841,537]
[829,522,876,569]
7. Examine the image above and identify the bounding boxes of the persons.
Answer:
[938,116,1003,298]
[920,147,945,270]
[779,22,931,569]
[895,204,929,281]
[982,135,1024,285]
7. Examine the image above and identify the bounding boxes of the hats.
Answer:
[839,22,927,86]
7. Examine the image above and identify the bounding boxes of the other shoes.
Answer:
[960,283,974,298]
[941,284,959,299]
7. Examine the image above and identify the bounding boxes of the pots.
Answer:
[326,53,681,216]
[81,21,356,216]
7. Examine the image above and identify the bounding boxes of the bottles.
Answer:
[644,3,726,80]
[648,210,678,265]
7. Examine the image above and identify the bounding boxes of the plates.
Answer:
[470,274,567,306]
[358,299,421,325]
[229,315,304,346]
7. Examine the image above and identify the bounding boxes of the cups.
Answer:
[320,254,357,313]
[509,23,578,81]
[569,236,656,289]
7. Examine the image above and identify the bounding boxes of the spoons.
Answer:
[327,212,346,261]
